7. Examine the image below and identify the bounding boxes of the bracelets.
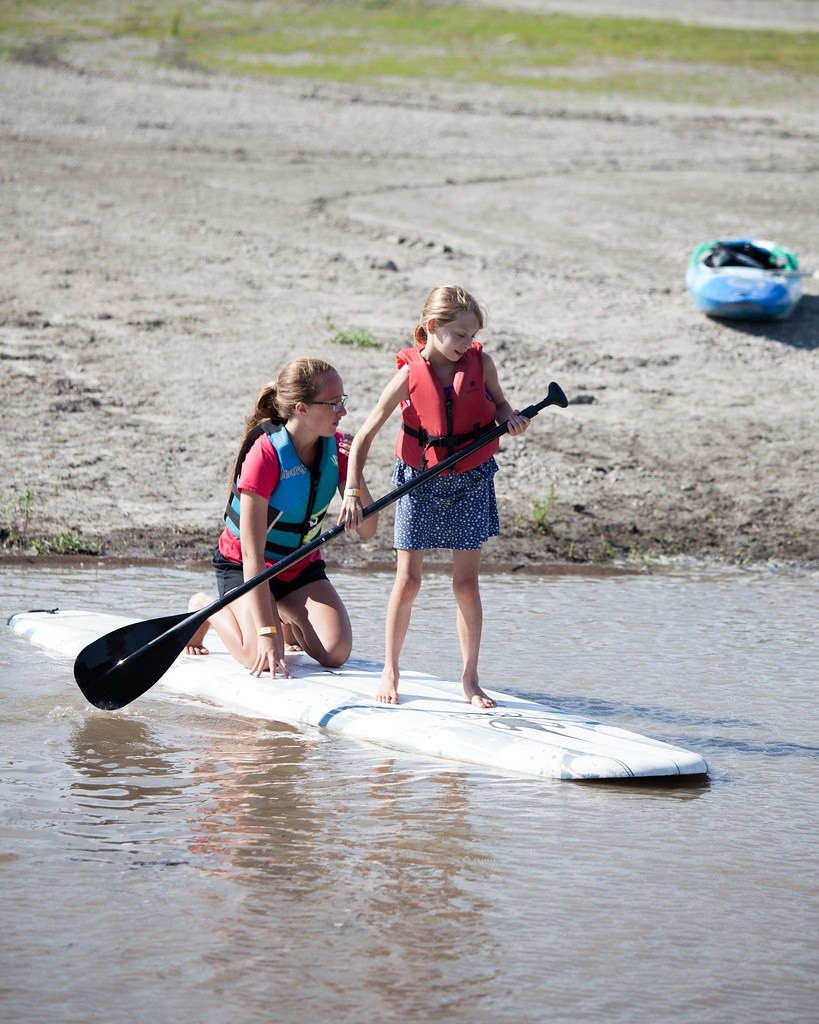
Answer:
[344,488,362,498]
[257,626,278,637]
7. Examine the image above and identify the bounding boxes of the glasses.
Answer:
[310,393,350,412]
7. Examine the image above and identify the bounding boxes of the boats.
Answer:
[685,240,803,320]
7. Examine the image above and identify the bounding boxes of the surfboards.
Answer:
[7,607,710,783]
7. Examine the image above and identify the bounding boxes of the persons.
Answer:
[336,286,531,707]
[182,359,376,680]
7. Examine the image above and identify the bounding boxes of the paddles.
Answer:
[73,381,569,711]
